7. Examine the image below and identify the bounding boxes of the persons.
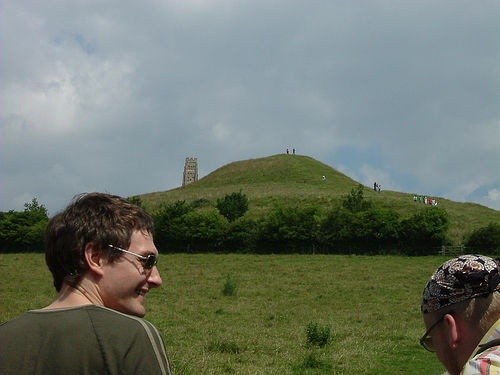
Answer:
[420,254,500,375]
[0,192,173,375]
[378,186,437,207]
[287,148,296,155]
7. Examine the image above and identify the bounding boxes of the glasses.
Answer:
[108,245,159,269]
[420,311,455,352]
